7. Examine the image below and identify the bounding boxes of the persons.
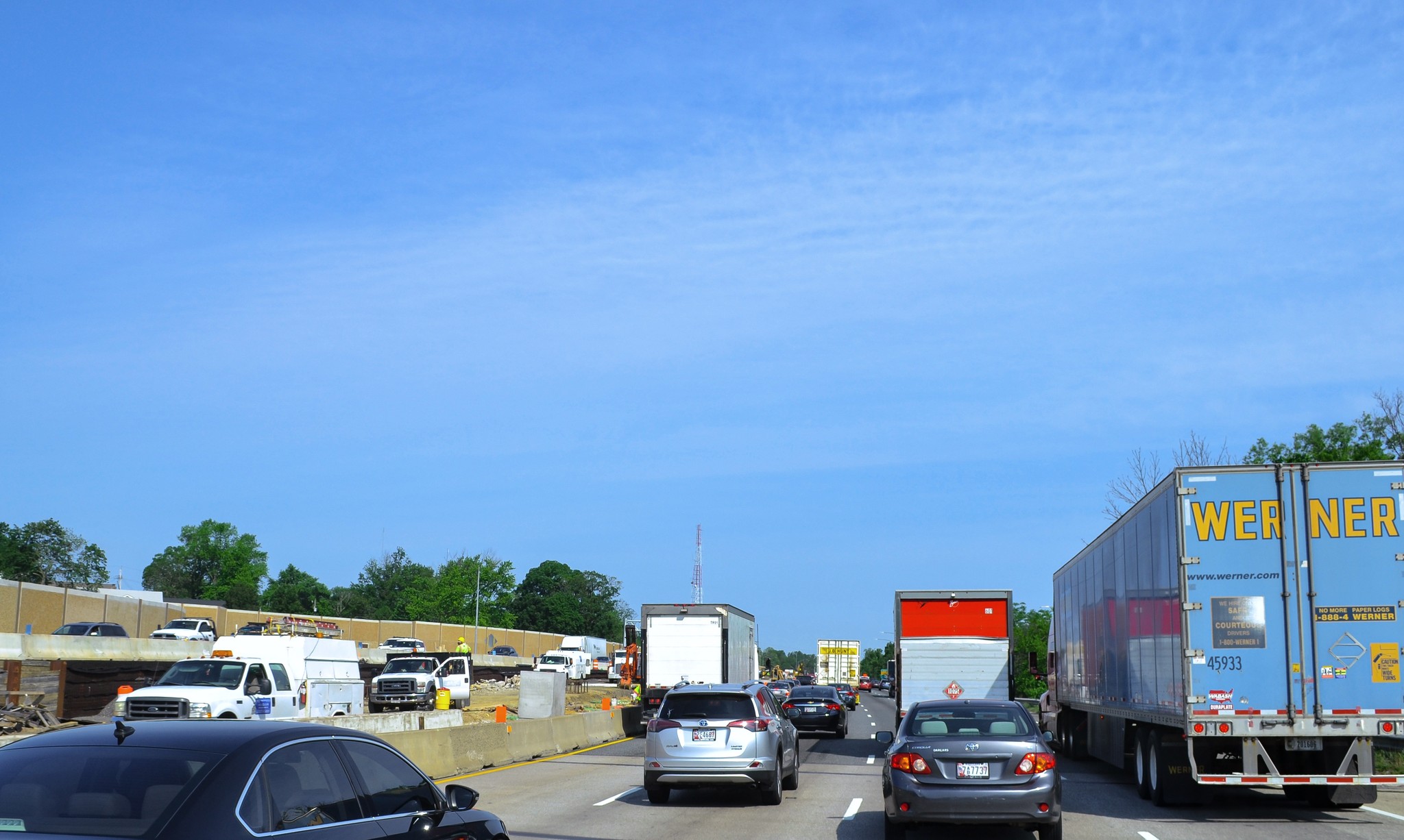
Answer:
[455,637,471,654]
[632,684,642,706]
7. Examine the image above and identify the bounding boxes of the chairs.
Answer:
[119,760,193,803]
[769,685,773,687]
[265,763,303,805]
[843,686,847,690]
[921,721,948,734]
[989,722,1016,734]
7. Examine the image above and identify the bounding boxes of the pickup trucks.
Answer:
[112,634,365,720]
[533,650,592,686]
[366,654,470,714]
[151,617,218,641]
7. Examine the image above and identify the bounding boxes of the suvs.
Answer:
[51,622,130,638]
[377,636,427,653]
[644,680,800,806]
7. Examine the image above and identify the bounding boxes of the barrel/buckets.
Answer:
[436,687,450,710]
[118,685,133,698]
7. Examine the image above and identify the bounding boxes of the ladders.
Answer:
[263,616,344,637]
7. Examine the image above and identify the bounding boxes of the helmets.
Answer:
[458,637,464,642]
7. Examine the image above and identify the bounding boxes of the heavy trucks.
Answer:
[885,589,1019,731]
[816,639,861,704]
[1028,459,1404,809]
[639,604,754,737]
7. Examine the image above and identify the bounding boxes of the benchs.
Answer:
[0,784,183,818]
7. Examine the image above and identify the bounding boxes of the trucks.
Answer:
[557,635,641,690]
[230,615,345,639]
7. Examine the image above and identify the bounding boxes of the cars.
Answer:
[827,683,858,711]
[0,720,510,840]
[765,682,791,704]
[874,699,1066,840]
[776,673,815,689]
[487,645,518,656]
[859,675,891,693]
[781,685,852,738]
